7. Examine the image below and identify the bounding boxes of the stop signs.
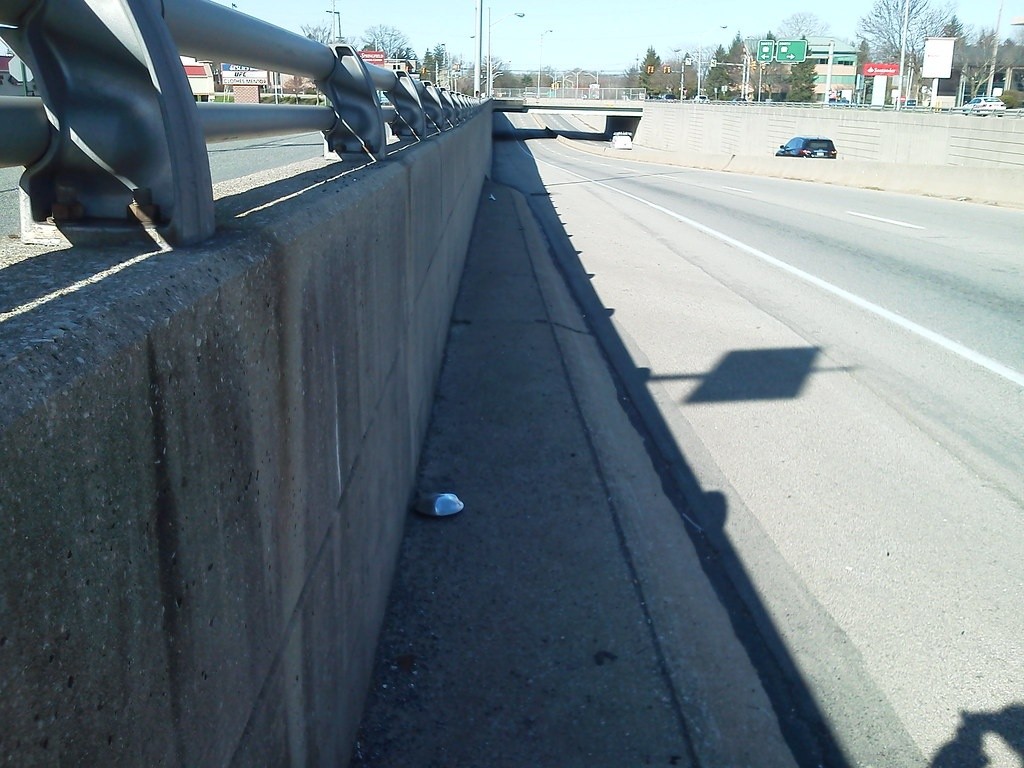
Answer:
[897,96,906,102]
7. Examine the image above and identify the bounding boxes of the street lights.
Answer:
[721,25,750,101]
[487,7,524,98]
[674,44,702,95]
[584,71,598,84]
[537,30,552,99]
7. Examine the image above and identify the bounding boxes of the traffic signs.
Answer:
[776,40,807,62]
[757,40,775,63]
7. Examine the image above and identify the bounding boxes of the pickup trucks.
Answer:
[693,95,710,104]
[660,94,677,101]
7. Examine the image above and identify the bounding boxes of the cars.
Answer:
[775,135,837,159]
[829,99,852,107]
[961,97,1006,117]
[609,136,633,150]
[896,96,916,105]
[376,89,391,105]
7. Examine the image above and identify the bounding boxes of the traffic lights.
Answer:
[557,83,561,89]
[663,65,671,74]
[647,65,655,74]
[551,83,555,90]
[422,67,426,74]
